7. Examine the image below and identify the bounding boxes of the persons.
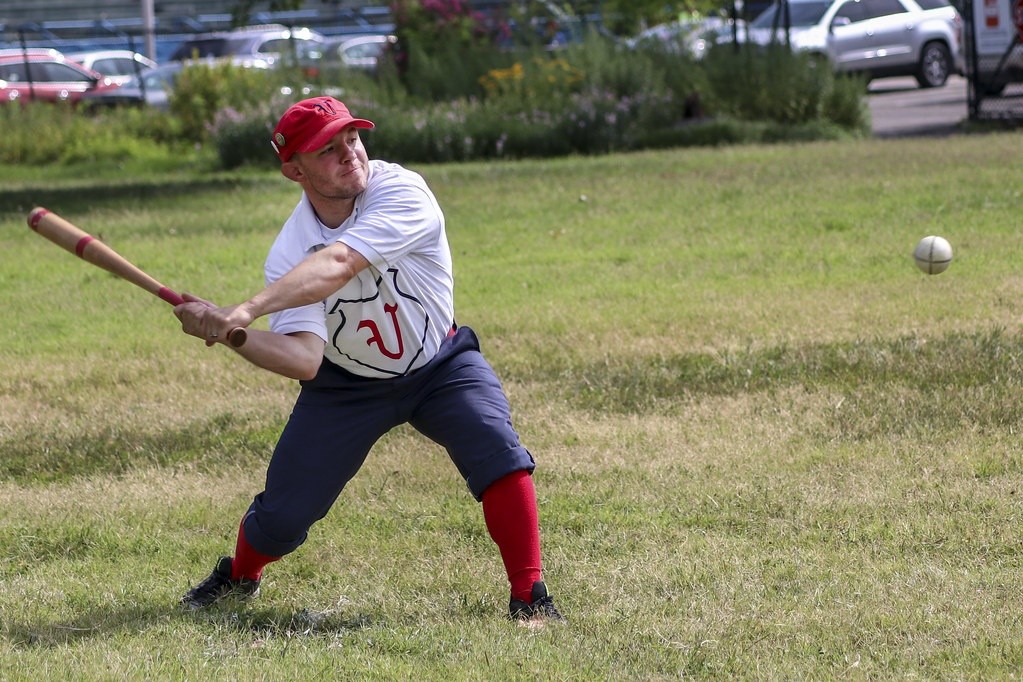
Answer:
[173,98,564,627]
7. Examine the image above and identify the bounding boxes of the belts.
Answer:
[447,326,457,338]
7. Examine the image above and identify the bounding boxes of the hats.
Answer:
[271,97,375,162]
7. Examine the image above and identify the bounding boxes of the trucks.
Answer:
[956,0,1023,96]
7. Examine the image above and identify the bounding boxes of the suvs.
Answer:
[0,49,125,109]
[63,51,160,84]
[169,27,324,64]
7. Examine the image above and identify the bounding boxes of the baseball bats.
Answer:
[25,205,249,349]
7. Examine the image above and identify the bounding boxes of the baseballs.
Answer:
[911,233,954,276]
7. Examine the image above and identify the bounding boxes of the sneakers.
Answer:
[504,581,562,625]
[176,556,262,610]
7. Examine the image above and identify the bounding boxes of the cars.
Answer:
[271,34,394,72]
[713,0,963,89]
[625,18,750,61]
[84,59,270,113]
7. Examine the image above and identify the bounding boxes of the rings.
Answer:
[210,335,217,337]
